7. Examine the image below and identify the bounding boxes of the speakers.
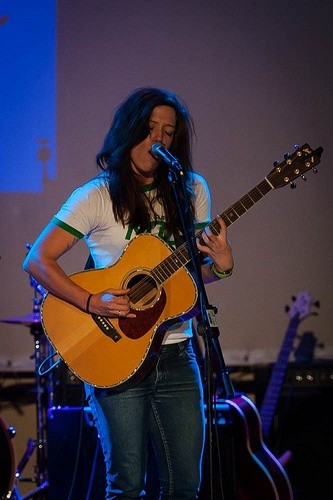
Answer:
[253,360,333,500]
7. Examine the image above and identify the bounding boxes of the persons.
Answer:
[22,87,235,500]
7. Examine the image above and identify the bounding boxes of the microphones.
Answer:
[152,142,182,172]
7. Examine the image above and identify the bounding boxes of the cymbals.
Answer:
[0,313,43,330]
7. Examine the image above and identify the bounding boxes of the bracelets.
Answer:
[86,294,92,312]
[210,263,233,279]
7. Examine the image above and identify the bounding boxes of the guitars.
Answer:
[257,290,322,443]
[200,306,294,499]
[39,140,325,393]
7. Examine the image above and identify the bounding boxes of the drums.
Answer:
[0,414,19,500]
